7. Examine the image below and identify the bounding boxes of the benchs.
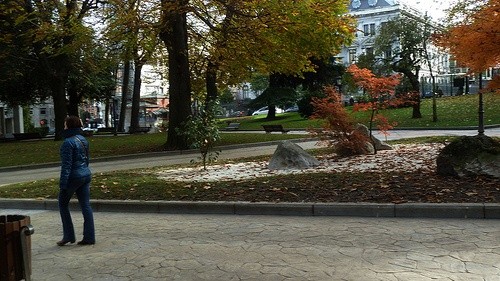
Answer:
[261,124,290,134]
[225,123,241,130]
[129,124,152,134]
[13,132,42,142]
[96,127,117,133]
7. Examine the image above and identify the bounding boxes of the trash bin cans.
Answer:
[0,214,35,281]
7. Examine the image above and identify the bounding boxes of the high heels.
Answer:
[77,239,96,245]
[56,236,76,245]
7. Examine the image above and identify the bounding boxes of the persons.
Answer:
[85,115,98,128]
[56,115,96,246]
